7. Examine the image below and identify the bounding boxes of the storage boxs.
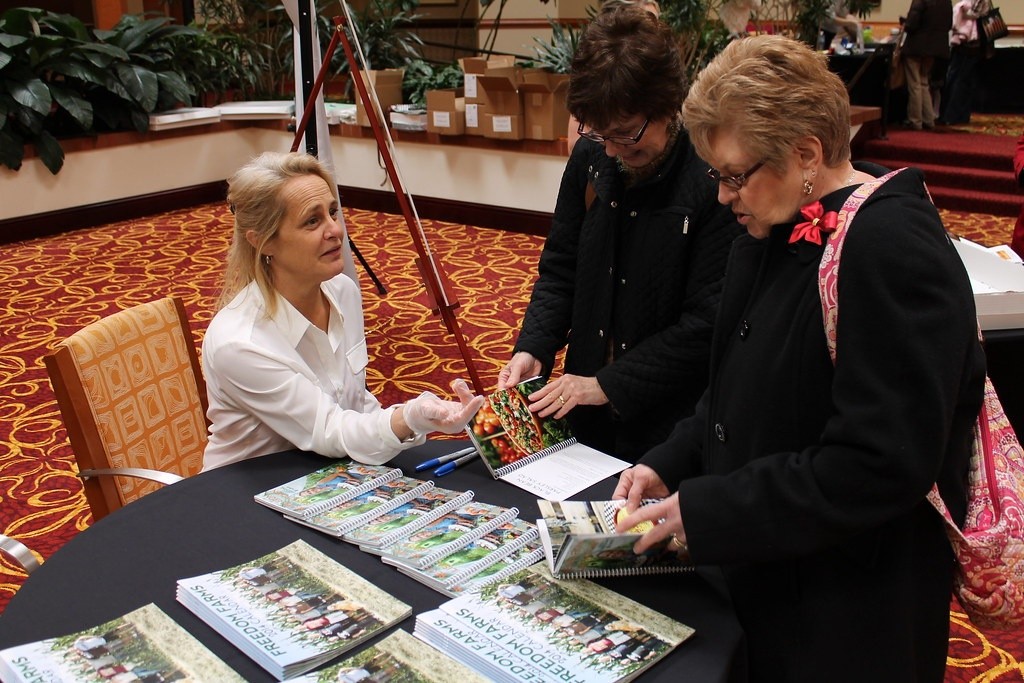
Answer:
[850,106,882,124]
[349,54,572,141]
[970,242,1024,330]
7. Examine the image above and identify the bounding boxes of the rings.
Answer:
[673,536,684,550]
[559,396,565,405]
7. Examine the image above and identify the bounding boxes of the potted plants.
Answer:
[175,0,430,109]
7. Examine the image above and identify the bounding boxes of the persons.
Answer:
[612,32,981,683]
[65,623,188,683]
[465,529,508,551]
[408,514,480,543]
[371,498,435,525]
[334,485,396,510]
[202,150,484,467]
[303,471,367,496]
[234,555,399,682]
[496,574,661,667]
[599,0,995,133]
[596,547,686,565]
[496,8,747,466]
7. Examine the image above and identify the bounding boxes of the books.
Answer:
[536,498,695,579]
[287,627,496,683]
[463,375,633,502]
[414,557,697,683]
[255,459,544,597]
[176,538,413,683]
[0,601,249,683]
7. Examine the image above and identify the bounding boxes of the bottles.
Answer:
[886,29,900,43]
[863,26,871,44]
[818,31,825,49]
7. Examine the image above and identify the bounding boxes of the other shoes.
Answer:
[901,122,935,130]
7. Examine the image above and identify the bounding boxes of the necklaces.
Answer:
[847,169,856,188]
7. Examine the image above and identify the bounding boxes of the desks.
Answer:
[0,440,754,683]
[824,42,909,125]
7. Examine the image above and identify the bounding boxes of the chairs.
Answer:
[44,298,214,523]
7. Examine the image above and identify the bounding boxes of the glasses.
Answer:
[578,114,652,146]
[708,157,769,190]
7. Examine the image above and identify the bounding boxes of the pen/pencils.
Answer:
[434,450,478,477]
[416,447,475,472]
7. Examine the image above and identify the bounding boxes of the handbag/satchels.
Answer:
[890,22,907,84]
[955,373,1024,631]
[974,0,1008,42]
